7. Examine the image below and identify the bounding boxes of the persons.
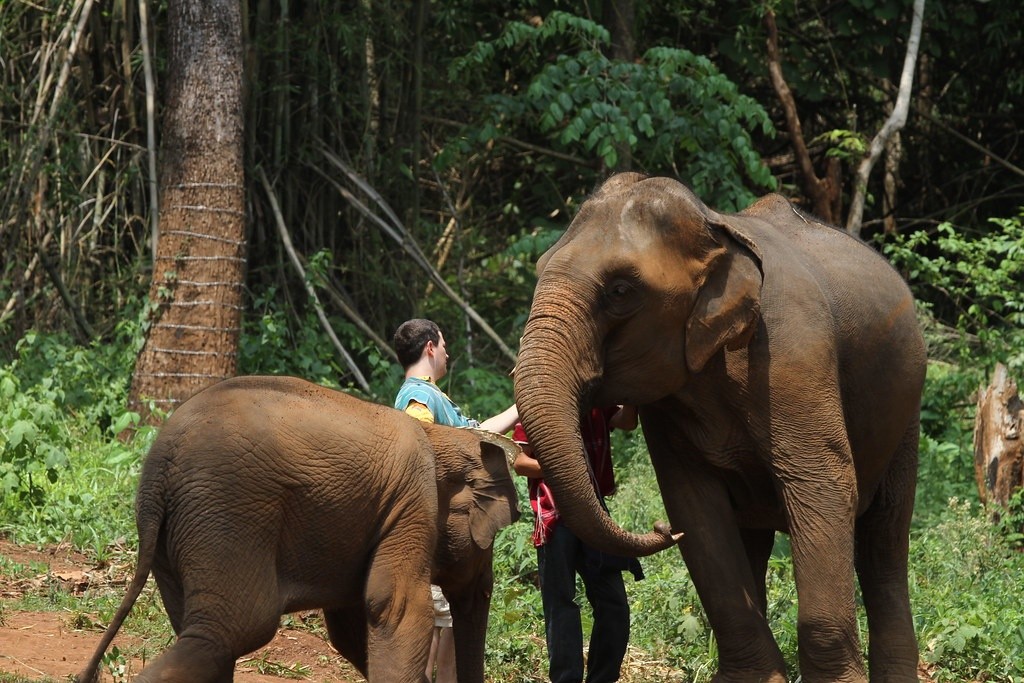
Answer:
[509,368,639,683]
[393,318,520,683]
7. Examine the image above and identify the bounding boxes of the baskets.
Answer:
[456,426,522,471]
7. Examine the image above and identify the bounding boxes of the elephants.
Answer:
[74,377,524,683]
[514,173,928,683]
